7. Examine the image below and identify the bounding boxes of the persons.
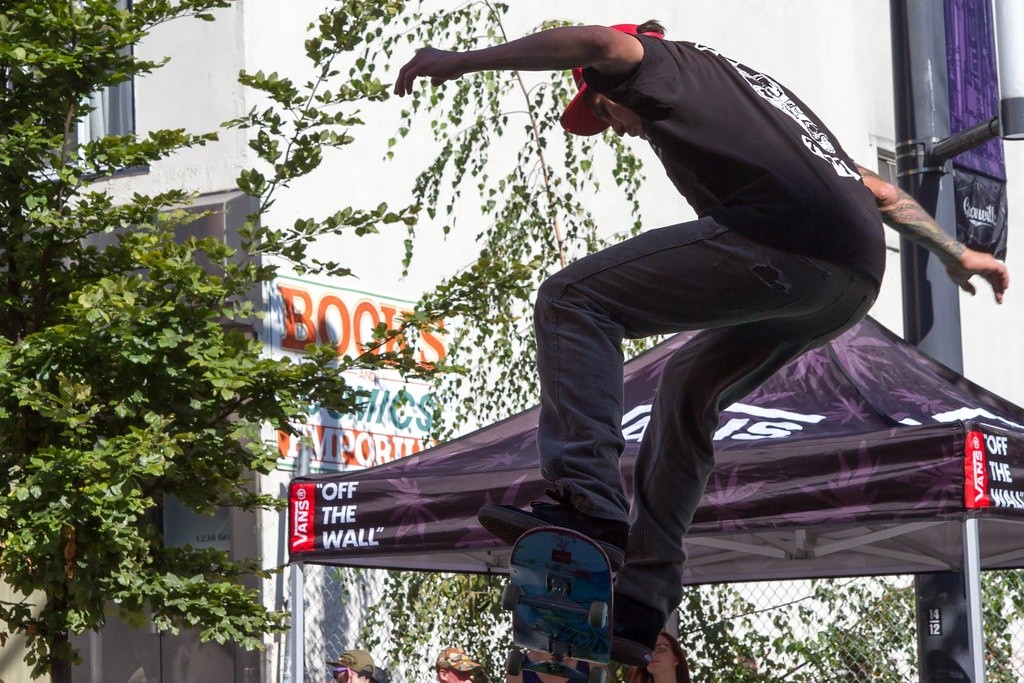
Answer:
[648,632,689,683]
[436,648,481,683]
[393,19,1010,668]
[327,650,390,683]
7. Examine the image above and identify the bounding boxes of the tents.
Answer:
[288,315,1023,683]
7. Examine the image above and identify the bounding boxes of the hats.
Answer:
[436,648,481,673]
[560,24,664,136]
[325,650,375,674]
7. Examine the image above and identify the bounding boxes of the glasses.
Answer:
[332,667,356,679]
[655,643,672,654]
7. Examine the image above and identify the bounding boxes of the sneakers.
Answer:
[609,590,665,666]
[478,485,629,571]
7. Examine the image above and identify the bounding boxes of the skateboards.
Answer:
[500,526,615,683]
